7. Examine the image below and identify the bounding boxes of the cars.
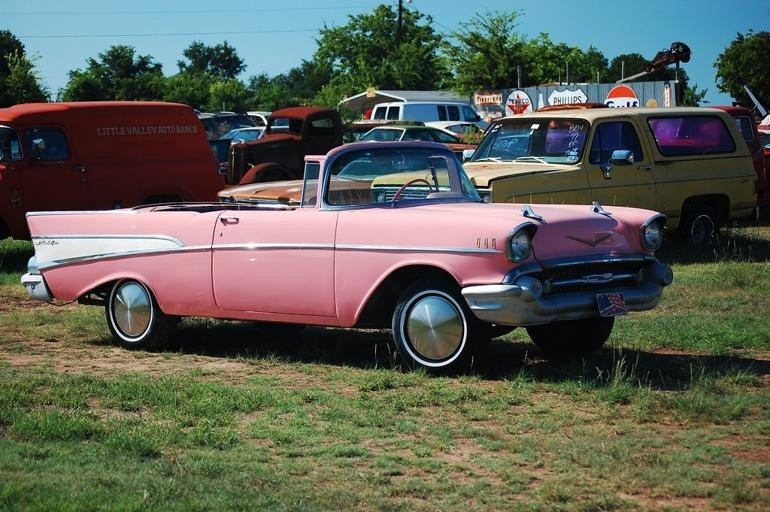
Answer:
[19,140,674,377]
